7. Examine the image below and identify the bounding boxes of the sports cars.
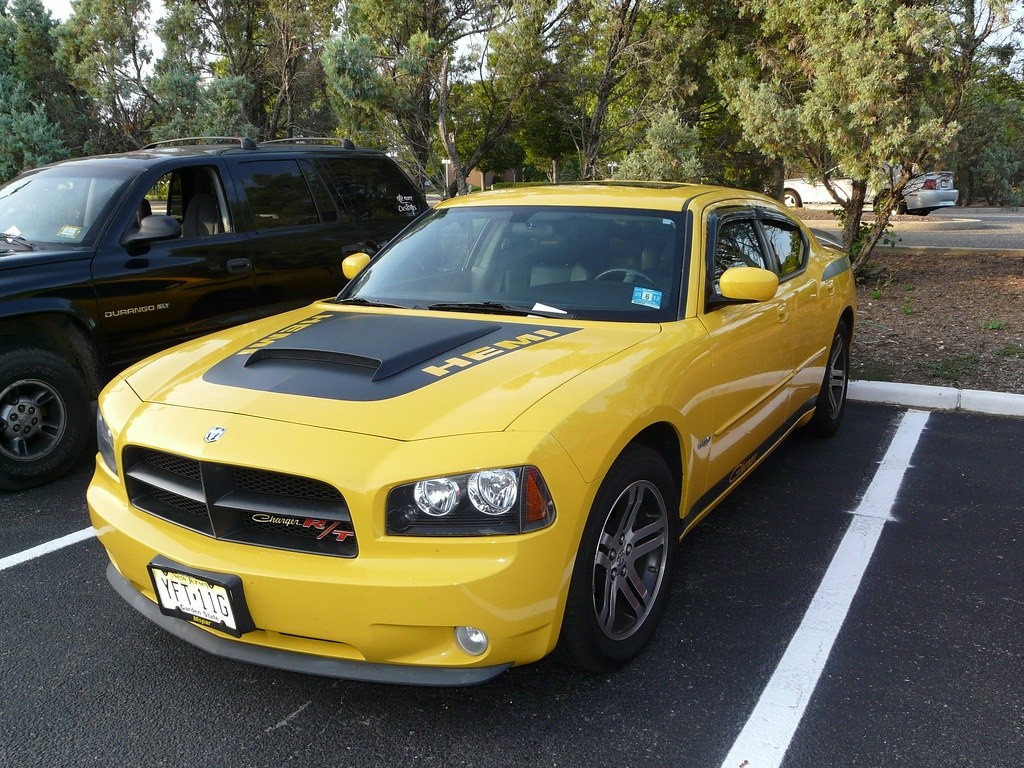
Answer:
[86,179,859,688]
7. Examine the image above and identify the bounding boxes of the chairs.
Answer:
[505,224,590,292]
[180,191,223,237]
[87,187,151,232]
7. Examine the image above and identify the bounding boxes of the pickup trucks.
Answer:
[784,159,960,216]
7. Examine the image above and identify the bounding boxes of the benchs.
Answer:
[562,228,676,283]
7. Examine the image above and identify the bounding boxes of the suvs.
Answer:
[0,135,433,493]
